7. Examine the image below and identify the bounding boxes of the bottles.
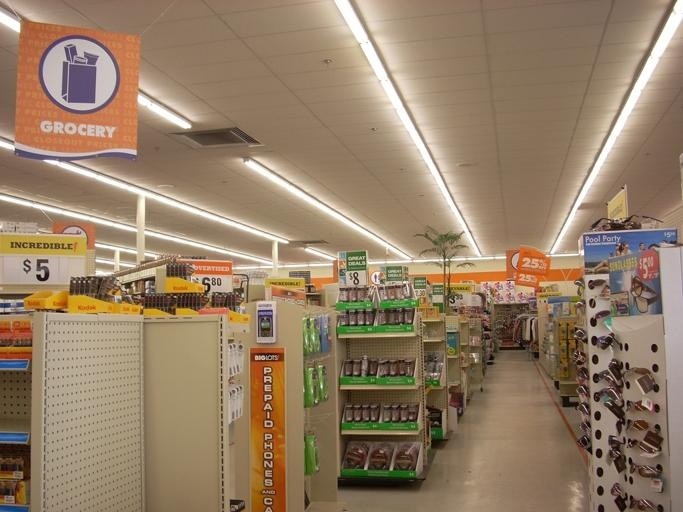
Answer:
[337,283,420,422]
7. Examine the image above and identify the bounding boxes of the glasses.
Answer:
[632,278,657,313]
[623,367,656,386]
[628,439,655,453]
[627,399,648,411]
[630,498,659,511]
[630,463,662,478]
[627,419,657,431]
[575,278,624,497]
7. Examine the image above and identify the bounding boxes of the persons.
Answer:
[584,238,659,276]
